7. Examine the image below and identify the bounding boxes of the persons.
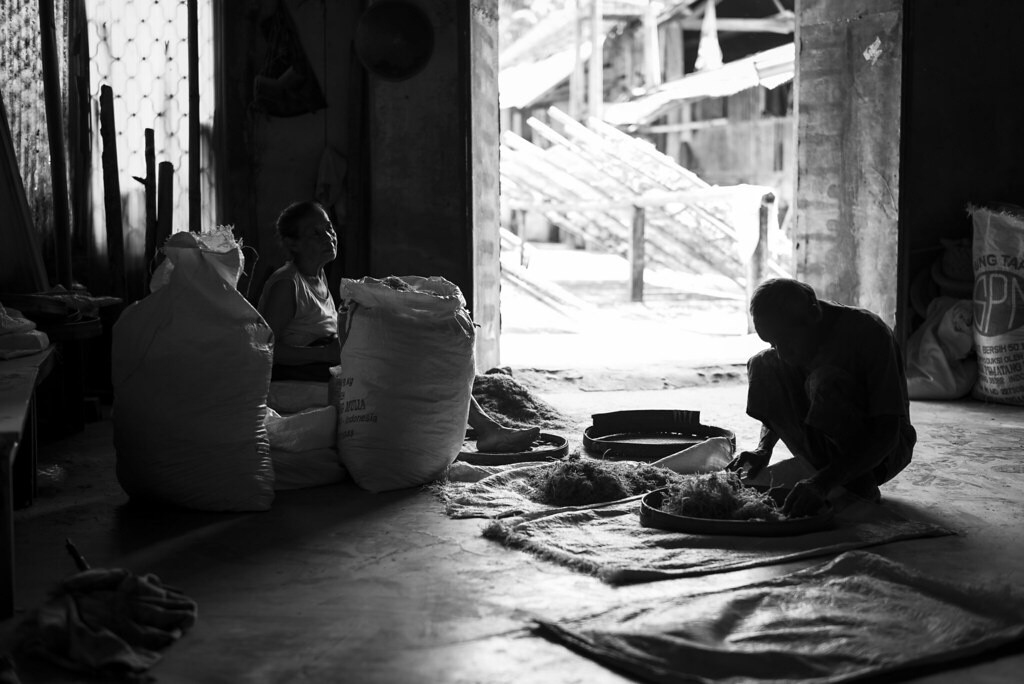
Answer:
[257,200,344,382]
[723,279,916,520]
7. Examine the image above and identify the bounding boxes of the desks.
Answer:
[0,344,56,618]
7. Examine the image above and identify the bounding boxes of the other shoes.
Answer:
[826,485,880,509]
